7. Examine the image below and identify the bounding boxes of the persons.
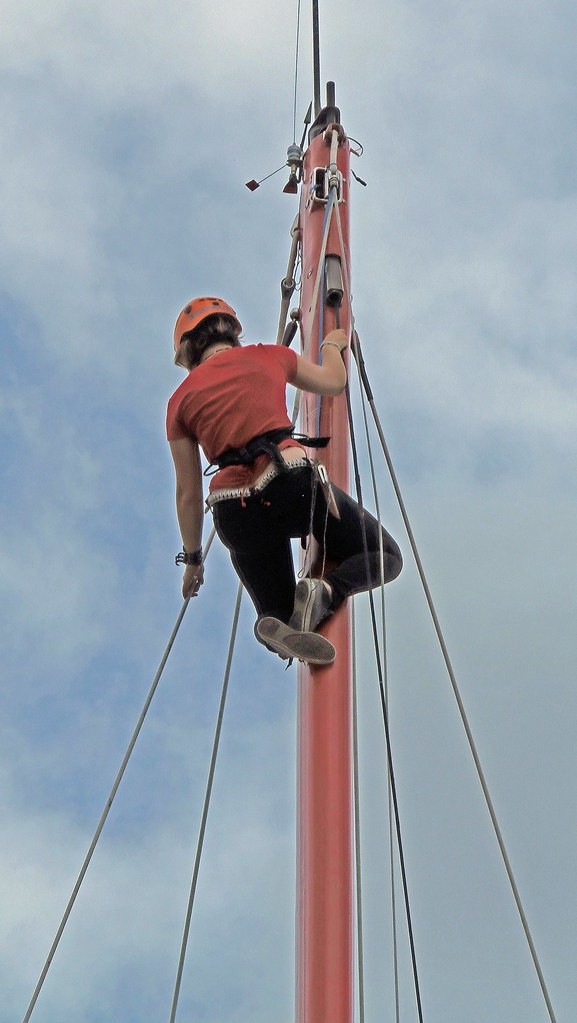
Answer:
[165,295,403,666]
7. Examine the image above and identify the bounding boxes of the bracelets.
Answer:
[318,341,340,352]
[174,545,203,567]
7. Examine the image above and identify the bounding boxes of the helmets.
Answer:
[173,296,244,368]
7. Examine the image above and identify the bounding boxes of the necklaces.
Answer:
[204,347,230,361]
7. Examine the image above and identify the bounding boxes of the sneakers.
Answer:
[256,616,338,672]
[287,577,335,633]
